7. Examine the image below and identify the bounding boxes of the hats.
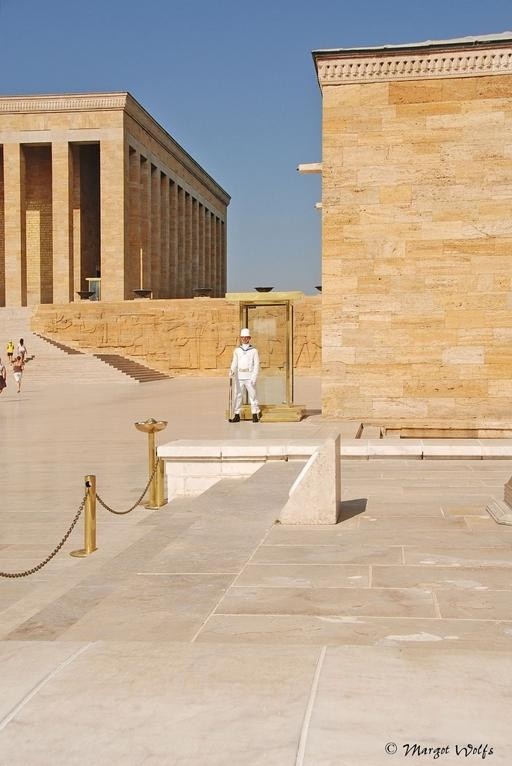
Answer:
[240,329,250,337]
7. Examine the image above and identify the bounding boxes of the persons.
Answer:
[0,358,7,394]
[12,356,24,393]
[6,339,14,366]
[228,328,260,423]
[17,338,27,365]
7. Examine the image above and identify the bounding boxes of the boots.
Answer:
[229,414,240,422]
[253,414,258,423]
